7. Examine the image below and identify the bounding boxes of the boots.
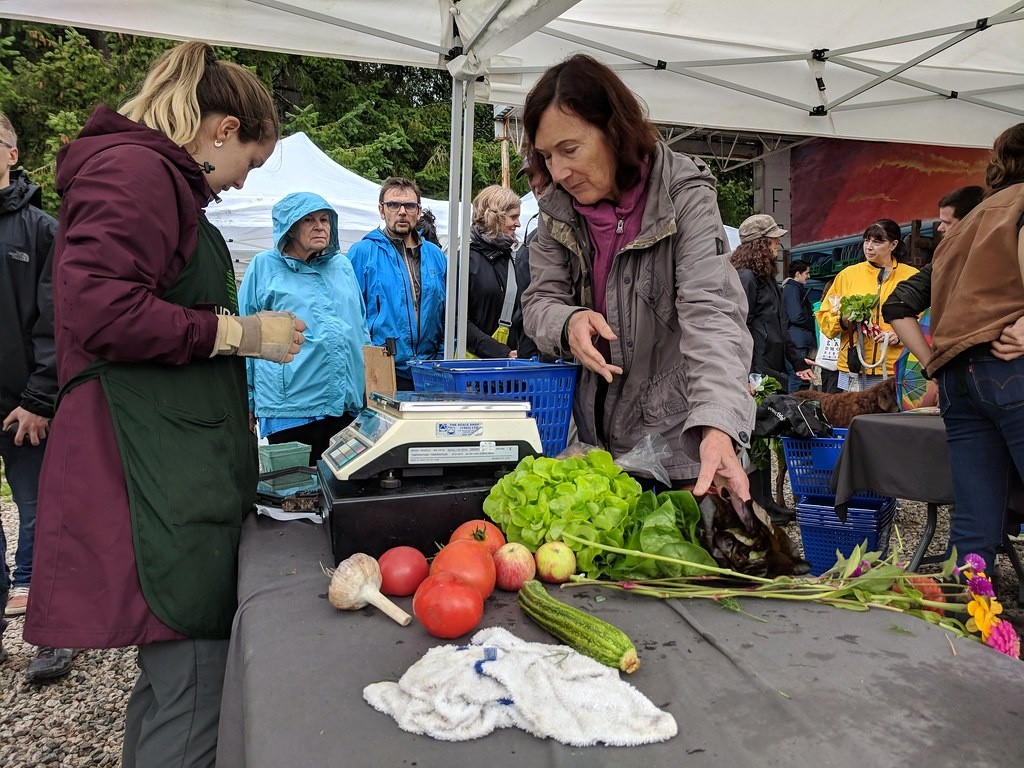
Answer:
[762,469,796,520]
[747,468,789,526]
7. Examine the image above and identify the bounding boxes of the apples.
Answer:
[492,542,535,592]
[536,541,577,585]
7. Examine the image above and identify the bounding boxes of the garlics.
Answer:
[319,552,412,627]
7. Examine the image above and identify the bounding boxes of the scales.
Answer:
[321,392,543,489]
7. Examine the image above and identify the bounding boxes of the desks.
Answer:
[214,493,1024,768]
[834,405,1024,590]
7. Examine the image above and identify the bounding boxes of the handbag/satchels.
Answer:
[451,327,509,366]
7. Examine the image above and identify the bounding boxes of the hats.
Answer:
[515,155,538,181]
[738,214,788,243]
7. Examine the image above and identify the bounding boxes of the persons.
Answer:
[453,184,537,358]
[937,185,985,237]
[881,123,1024,618]
[816,217,921,392]
[346,177,447,391]
[729,214,817,525]
[238,192,371,465]
[782,259,815,393]
[23,41,306,768]
[520,54,757,522]
[0,112,79,682]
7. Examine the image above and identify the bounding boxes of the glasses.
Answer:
[379,200,421,213]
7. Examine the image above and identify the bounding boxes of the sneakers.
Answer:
[24,644,81,681]
[4,586,30,615]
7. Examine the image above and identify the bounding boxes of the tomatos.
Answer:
[376,519,505,639]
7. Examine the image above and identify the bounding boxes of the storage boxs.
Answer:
[259,440,311,485]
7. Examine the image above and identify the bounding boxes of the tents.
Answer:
[201,132,474,274]
[0,0,1024,361]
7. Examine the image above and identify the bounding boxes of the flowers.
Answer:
[558,524,1021,661]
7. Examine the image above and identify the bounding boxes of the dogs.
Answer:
[774,378,895,510]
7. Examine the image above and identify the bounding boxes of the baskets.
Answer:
[779,424,893,508]
[407,353,580,461]
[795,496,895,578]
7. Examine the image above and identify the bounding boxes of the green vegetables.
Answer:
[748,375,787,471]
[483,450,813,587]
